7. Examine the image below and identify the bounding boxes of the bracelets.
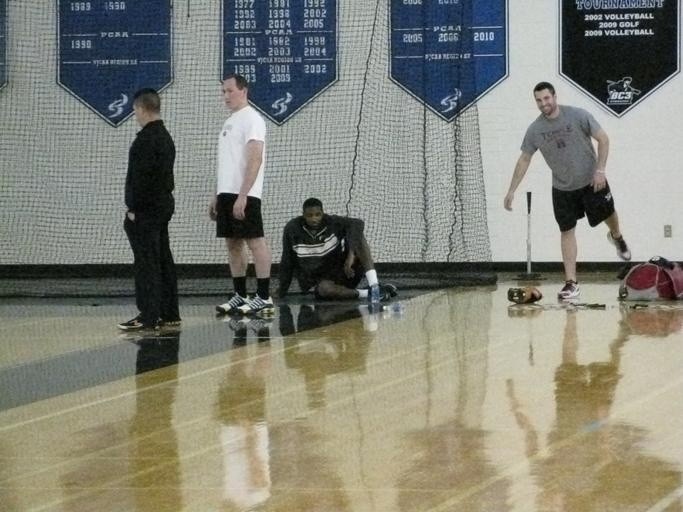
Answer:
[594,170,607,174]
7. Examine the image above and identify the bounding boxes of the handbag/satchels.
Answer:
[619,256,683,300]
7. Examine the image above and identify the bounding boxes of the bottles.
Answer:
[370,281,380,305]
[381,303,404,314]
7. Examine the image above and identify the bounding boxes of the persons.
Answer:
[208,74,274,314]
[503,83,631,299]
[118,86,182,331]
[273,199,399,302]
[215,315,271,510]
[122,333,181,511]
[505,300,631,512]
[280,304,407,412]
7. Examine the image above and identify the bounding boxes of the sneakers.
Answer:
[216,292,275,315]
[364,283,400,302]
[607,231,631,260]
[117,312,182,331]
[557,280,582,299]
